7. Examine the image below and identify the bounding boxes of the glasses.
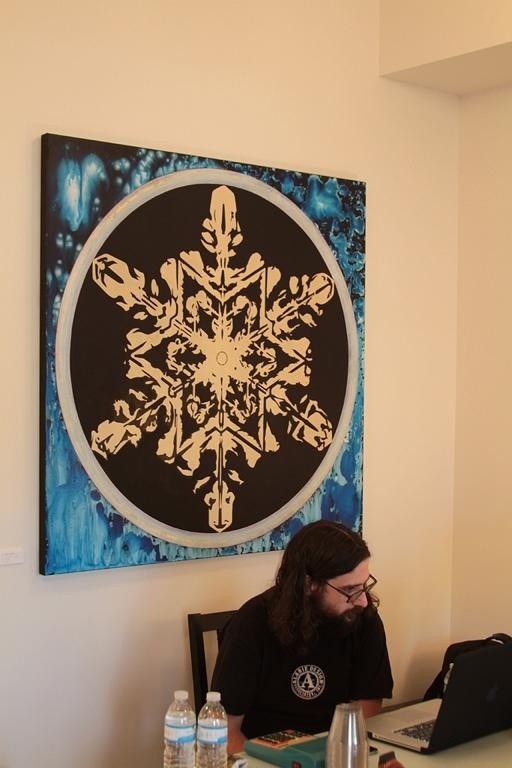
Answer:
[324,574,377,603]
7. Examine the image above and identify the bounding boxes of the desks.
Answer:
[227,697,512,768]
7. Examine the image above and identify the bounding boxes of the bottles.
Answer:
[325,705,367,767]
[162,690,229,767]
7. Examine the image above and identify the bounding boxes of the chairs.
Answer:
[189,610,238,712]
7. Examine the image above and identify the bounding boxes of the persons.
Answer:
[204,518,395,756]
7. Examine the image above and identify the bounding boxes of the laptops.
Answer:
[367,642,512,755]
